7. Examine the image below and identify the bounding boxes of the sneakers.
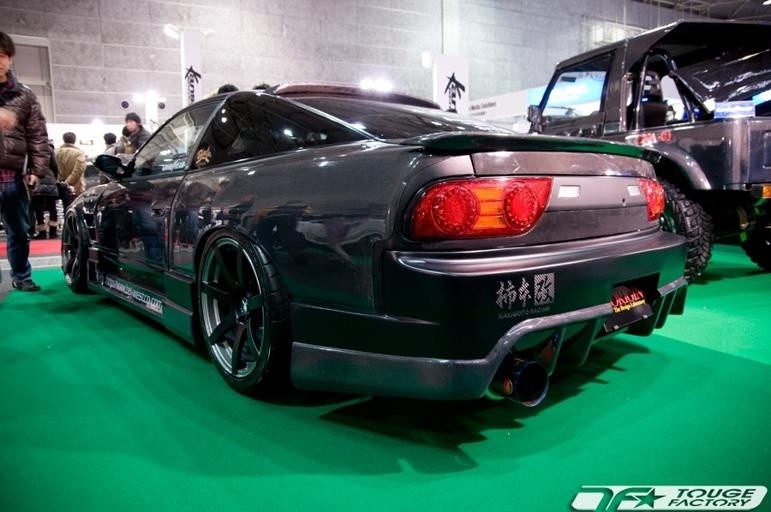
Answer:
[11,277,38,291]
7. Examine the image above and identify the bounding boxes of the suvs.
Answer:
[526,13,770,285]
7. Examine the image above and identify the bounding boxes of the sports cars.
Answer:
[56,81,692,410]
